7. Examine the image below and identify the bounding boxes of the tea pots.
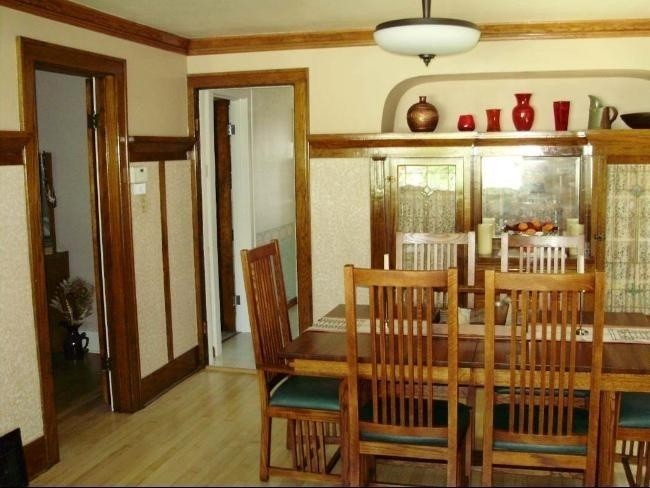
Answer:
[587,95,618,130]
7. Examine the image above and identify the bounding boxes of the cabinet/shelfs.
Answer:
[367,129,650,312]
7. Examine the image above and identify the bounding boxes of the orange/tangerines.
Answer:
[518,221,552,235]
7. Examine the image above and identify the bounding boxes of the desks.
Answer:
[288,299,649,487]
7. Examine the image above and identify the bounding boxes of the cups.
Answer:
[476,223,492,255]
[457,114,476,132]
[569,223,584,257]
[566,217,578,235]
[481,218,495,236]
[553,100,570,131]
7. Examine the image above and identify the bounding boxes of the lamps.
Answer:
[371,0,481,67]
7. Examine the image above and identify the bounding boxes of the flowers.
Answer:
[506,220,559,236]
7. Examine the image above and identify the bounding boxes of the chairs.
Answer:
[477,263,606,487]
[394,230,477,320]
[239,239,344,484]
[342,262,475,487]
[498,230,588,327]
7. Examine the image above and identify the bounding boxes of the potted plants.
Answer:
[49,277,96,356]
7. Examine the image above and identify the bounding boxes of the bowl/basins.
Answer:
[620,111,649,129]
